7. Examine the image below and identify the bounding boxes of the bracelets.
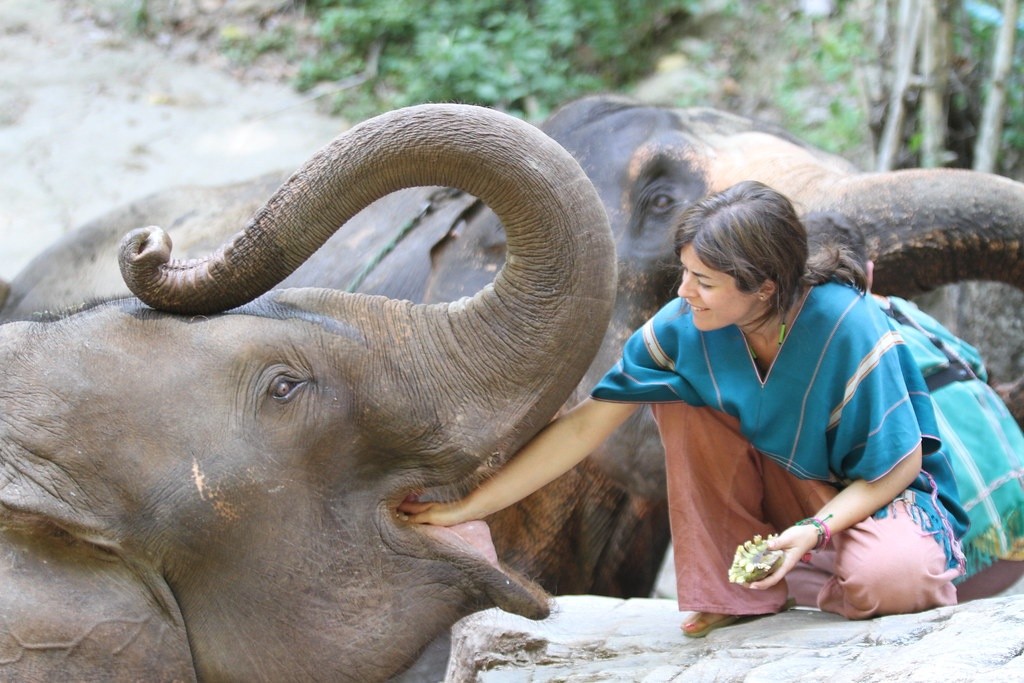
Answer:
[794,516,830,563]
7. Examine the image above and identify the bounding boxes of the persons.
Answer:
[785,210,1024,607]
[398,180,972,638]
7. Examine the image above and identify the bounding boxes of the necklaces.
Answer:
[743,308,786,378]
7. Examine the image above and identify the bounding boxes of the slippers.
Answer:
[683,593,798,638]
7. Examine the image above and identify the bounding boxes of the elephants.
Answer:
[1,96,1024,681]
